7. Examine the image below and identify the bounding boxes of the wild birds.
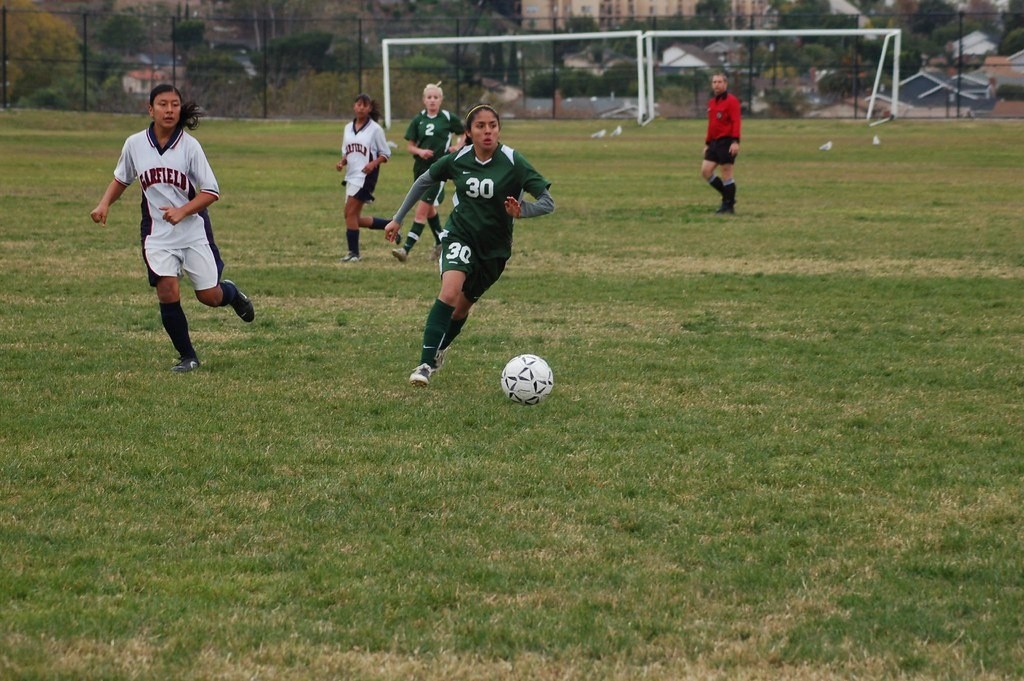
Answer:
[818,141,833,151]
[872,135,881,145]
[590,124,623,138]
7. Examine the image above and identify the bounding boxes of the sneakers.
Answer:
[223,279,256,322]
[336,251,360,262]
[429,242,443,261]
[409,363,432,388]
[171,357,200,372]
[434,350,447,371]
[395,221,402,245]
[392,247,407,261]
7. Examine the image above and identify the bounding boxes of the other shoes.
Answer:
[716,198,735,213]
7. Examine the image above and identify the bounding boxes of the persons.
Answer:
[336,94,405,262]
[391,83,467,262]
[701,74,742,214]
[384,103,556,386]
[89,83,255,373]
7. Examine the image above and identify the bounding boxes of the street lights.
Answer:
[955,4,969,120]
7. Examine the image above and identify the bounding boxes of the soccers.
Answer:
[500,353,555,408]
